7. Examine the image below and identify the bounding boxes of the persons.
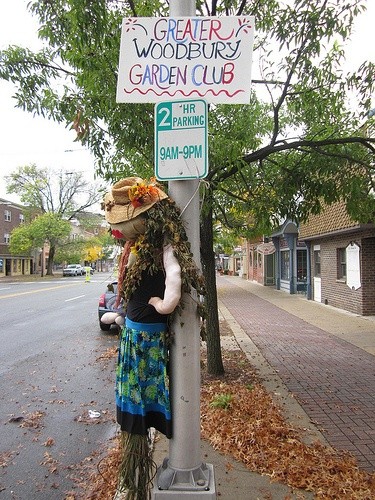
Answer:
[100,176,183,500]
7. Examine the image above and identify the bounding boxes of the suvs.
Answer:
[62,264,84,277]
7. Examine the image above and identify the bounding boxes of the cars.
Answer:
[82,266,94,276]
[98,281,125,330]
